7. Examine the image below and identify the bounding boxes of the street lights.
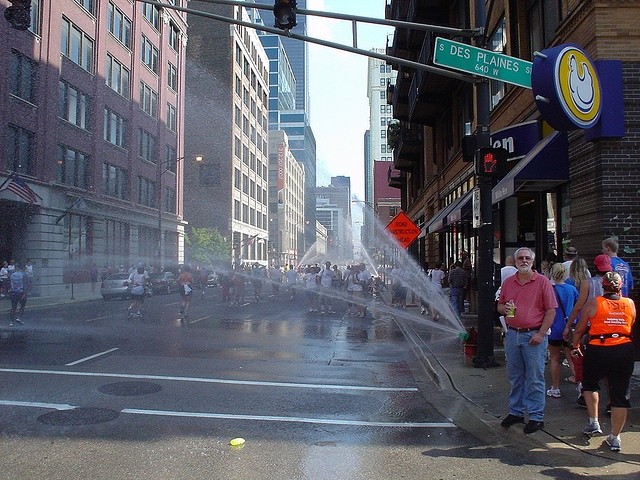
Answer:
[352,201,380,278]
[156,154,204,265]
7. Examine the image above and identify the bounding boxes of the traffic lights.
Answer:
[272,0,298,30]
[3,0,32,31]
[473,146,508,178]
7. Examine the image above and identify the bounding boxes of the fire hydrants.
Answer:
[457,326,479,367]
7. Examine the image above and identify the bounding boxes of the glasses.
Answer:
[518,256,531,261]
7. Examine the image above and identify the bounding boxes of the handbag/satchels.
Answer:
[362,283,370,292]
[571,354,584,381]
[179,284,193,296]
[568,324,575,341]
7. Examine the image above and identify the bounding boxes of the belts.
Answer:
[591,333,630,339]
[505,323,540,332]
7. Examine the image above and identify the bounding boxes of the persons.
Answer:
[428,260,445,323]
[25,260,33,297]
[177,265,192,317]
[303,266,319,312]
[353,266,364,318]
[563,247,591,281]
[401,263,410,310]
[601,238,633,297]
[343,265,351,278]
[345,269,354,316]
[136,262,151,310]
[496,247,558,433]
[317,261,336,314]
[563,254,619,412]
[546,262,579,398]
[8,260,15,292]
[449,262,465,318]
[501,256,518,283]
[416,262,430,316]
[565,257,590,384]
[357,263,372,318]
[573,272,636,451]
[391,265,401,307]
[89,263,125,292]
[198,262,299,308]
[0,261,9,298]
[127,267,152,318]
[332,265,342,290]
[10,264,28,326]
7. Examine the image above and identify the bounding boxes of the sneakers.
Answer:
[561,359,570,367]
[546,386,561,398]
[582,419,603,437]
[606,399,632,413]
[576,396,587,409]
[606,436,621,451]
[501,414,524,427]
[16,317,25,326]
[523,420,543,433]
[9,321,16,327]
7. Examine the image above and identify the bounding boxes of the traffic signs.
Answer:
[433,36,534,89]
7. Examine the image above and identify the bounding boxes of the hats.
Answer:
[565,247,577,255]
[594,255,613,271]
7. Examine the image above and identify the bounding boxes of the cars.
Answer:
[206,267,222,287]
[147,271,180,295]
[99,272,154,301]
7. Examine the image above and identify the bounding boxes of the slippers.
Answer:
[563,376,577,384]
